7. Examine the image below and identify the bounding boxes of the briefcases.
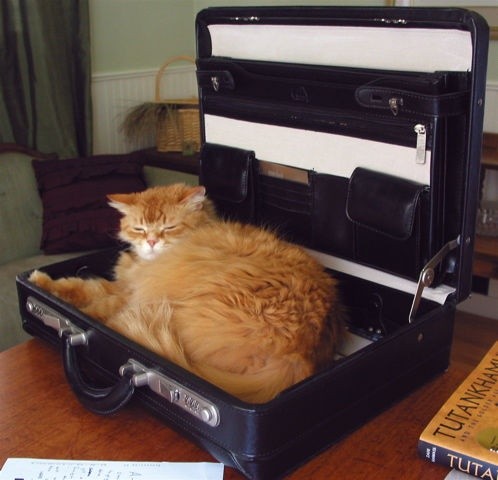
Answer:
[17,7,490,480]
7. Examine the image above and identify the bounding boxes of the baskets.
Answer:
[150,55,205,153]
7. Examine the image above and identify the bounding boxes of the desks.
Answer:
[131,147,201,189]
[0,311,498,479]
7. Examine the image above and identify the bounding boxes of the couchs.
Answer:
[1,142,199,353]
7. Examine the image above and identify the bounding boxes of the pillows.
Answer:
[31,151,148,255]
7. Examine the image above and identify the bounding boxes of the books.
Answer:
[417,341,498,480]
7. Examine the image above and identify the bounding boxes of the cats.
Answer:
[26,182,350,405]
